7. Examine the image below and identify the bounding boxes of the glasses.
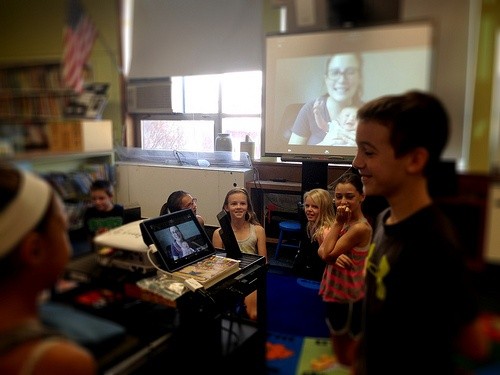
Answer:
[186,198,198,209]
[171,230,179,234]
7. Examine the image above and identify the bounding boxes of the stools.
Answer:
[273,220,301,260]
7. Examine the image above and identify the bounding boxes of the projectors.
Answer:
[93,219,160,269]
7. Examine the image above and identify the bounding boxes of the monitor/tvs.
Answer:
[216,210,243,261]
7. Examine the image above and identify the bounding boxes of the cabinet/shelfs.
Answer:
[117,163,253,227]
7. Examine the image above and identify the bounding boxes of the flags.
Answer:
[60,0,98,94]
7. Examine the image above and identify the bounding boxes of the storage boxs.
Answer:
[46,120,113,153]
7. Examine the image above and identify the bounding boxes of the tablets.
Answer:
[139,208,216,273]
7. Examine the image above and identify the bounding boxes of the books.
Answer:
[0,69,66,119]
[40,163,115,200]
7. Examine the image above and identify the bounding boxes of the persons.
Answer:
[303,189,336,246]
[180,242,194,256]
[288,52,368,146]
[0,165,97,375]
[169,226,204,261]
[212,189,267,319]
[330,107,359,147]
[352,91,486,375]
[84,179,127,251]
[160,191,204,229]
[318,173,373,366]
